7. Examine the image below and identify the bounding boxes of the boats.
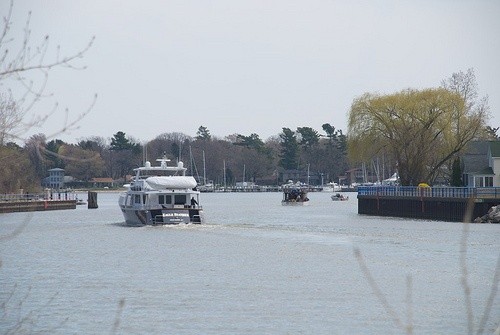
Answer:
[118,154,206,226]
[282,182,310,206]
[331,193,349,201]
